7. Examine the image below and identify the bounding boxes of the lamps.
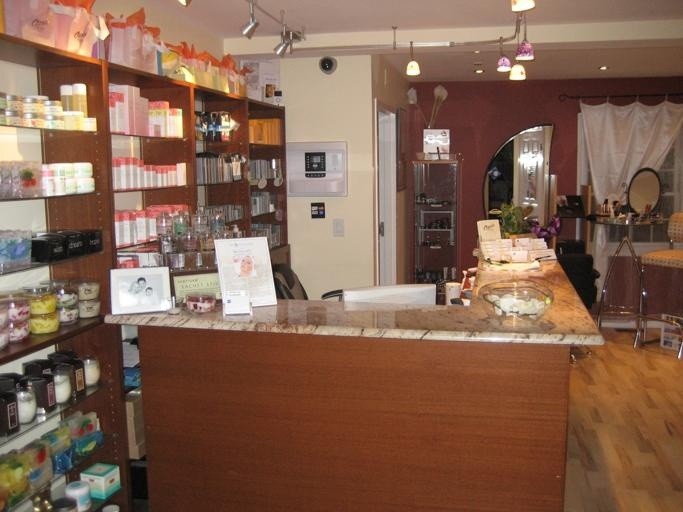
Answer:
[406,41,421,76]
[176,0,193,7]
[496,1,537,81]
[241,0,305,59]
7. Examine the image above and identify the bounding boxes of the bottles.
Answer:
[415,196,476,305]
[0,81,103,437]
[598,197,660,224]
[53,479,91,512]
[155,205,232,269]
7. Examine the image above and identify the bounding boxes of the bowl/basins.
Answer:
[184,290,217,314]
[479,278,553,322]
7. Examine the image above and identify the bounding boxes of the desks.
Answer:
[591,216,666,341]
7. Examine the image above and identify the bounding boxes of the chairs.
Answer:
[272,263,343,302]
[555,239,601,310]
[633,213,682,361]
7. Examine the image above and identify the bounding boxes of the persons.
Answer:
[118,277,158,307]
[235,255,257,278]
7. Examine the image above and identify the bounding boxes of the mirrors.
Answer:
[628,167,661,217]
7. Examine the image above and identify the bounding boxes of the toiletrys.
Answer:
[0,76,95,199]
[446,282,461,306]
[196,109,231,139]
[229,223,244,238]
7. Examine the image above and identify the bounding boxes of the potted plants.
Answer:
[488,200,540,247]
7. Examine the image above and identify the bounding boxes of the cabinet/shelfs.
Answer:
[0,33,289,511]
[404,153,465,295]
